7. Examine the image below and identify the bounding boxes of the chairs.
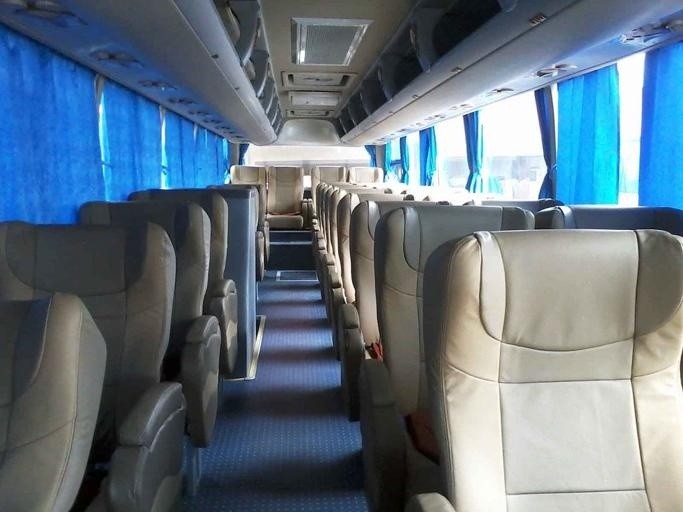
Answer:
[225,164,384,227]
